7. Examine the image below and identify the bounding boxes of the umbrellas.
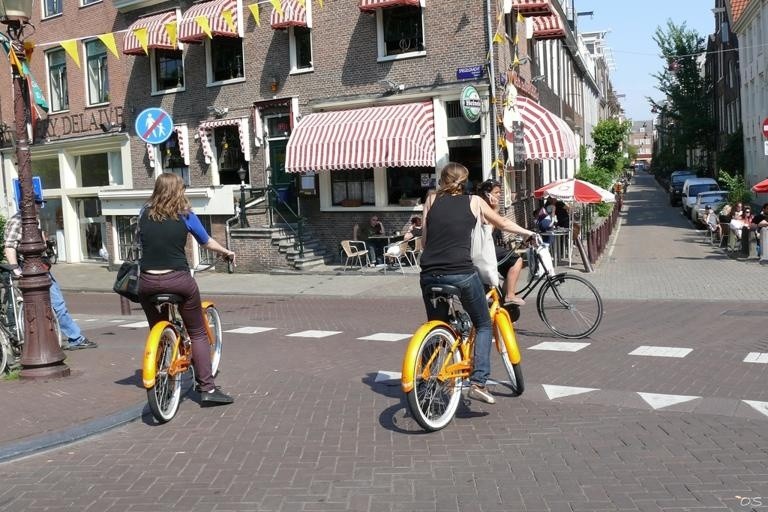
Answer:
[534,177,616,270]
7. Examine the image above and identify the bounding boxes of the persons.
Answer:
[3,192,98,351]
[420,166,537,404]
[532,198,569,258]
[359,216,385,267]
[750,178,768,193]
[475,179,526,307]
[136,173,237,405]
[702,202,768,240]
[403,217,424,265]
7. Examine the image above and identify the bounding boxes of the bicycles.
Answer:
[458,228,607,340]
[139,253,235,425]
[399,230,538,435]
[0,261,63,380]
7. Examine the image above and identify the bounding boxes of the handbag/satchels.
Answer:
[469,196,500,287]
[113,261,141,304]
[527,234,556,282]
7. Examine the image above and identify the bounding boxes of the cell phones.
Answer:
[485,193,493,198]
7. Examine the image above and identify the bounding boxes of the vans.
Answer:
[678,176,720,216]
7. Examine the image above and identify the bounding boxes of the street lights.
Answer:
[0,0,75,378]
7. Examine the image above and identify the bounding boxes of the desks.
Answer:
[364,233,402,269]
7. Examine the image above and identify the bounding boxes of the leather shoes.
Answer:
[200,388,234,404]
[70,338,97,352]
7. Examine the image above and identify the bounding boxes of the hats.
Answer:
[34,194,48,202]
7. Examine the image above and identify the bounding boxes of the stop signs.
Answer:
[761,118,767,138]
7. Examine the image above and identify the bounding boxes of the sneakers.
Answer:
[467,384,497,404]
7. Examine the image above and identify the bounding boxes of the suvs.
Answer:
[667,169,698,206]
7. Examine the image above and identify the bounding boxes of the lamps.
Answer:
[96,119,126,132]
[376,77,405,96]
[266,76,279,93]
[508,53,532,71]
[205,105,229,119]
[529,74,545,84]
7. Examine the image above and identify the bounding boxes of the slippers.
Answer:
[504,296,525,307]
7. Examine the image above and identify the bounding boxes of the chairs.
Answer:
[340,240,370,273]
[383,240,416,275]
[403,236,423,273]
[699,216,767,254]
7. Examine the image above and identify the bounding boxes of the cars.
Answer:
[689,190,729,227]
